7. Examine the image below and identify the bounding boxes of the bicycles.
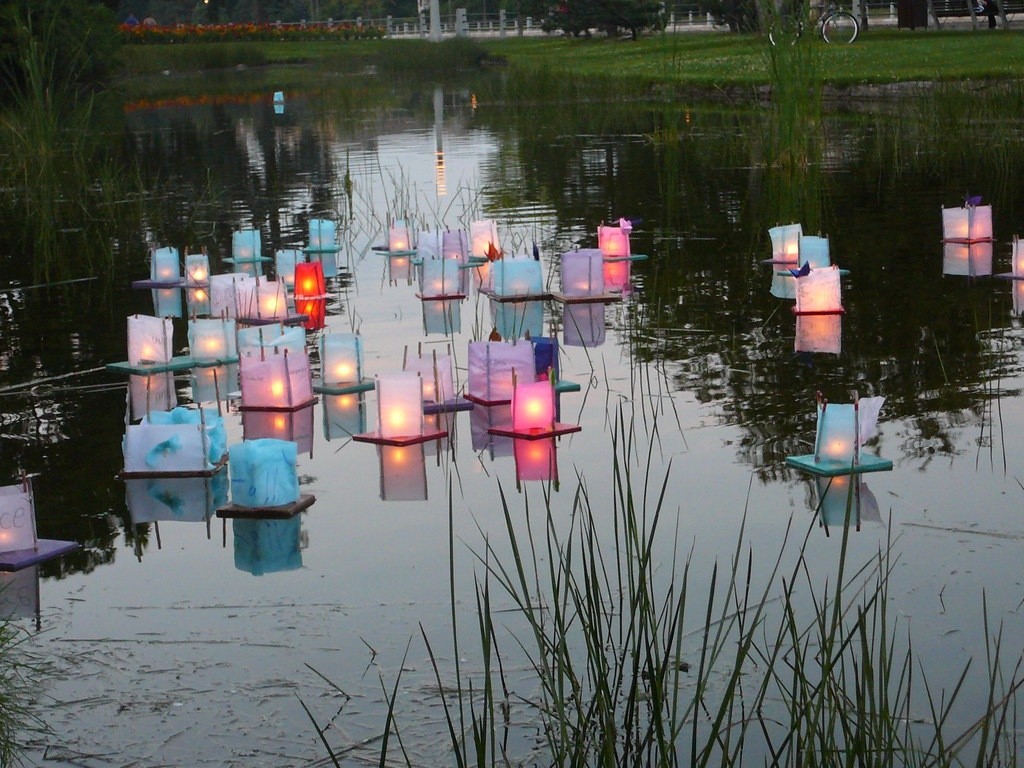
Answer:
[768,3,858,49]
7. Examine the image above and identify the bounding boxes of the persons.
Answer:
[143,15,156,26]
[124,12,139,25]
[810,0,840,28]
[980,0,998,28]
